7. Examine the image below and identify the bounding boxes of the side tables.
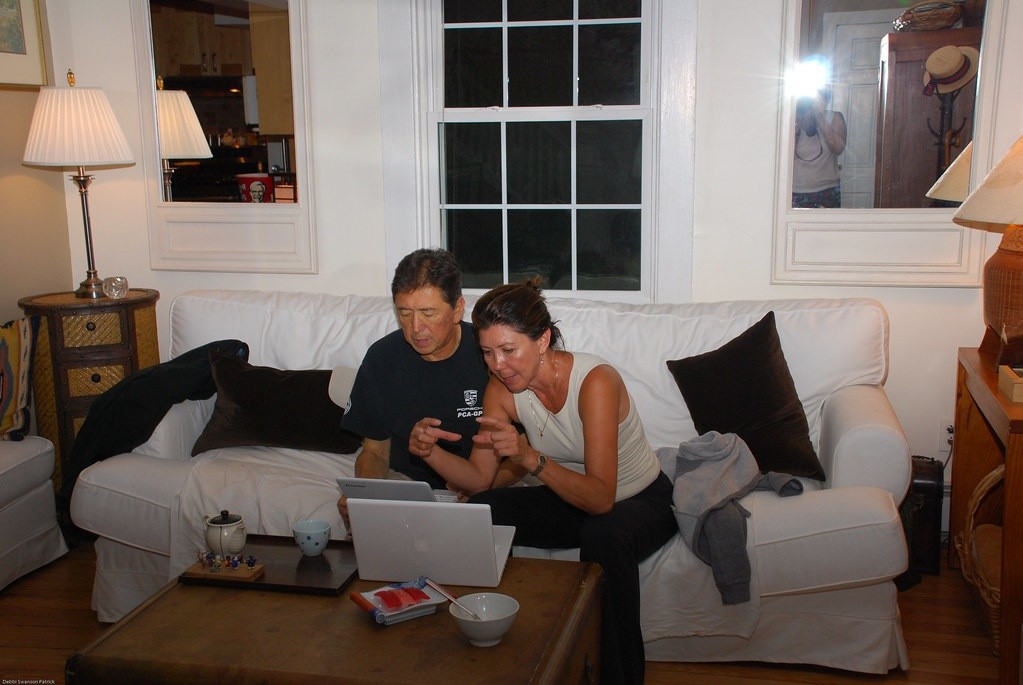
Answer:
[18,289,159,526]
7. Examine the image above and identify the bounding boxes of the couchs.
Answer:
[69,290,913,670]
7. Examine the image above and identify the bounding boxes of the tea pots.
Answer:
[202,509,247,560]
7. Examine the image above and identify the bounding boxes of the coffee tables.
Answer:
[65,543,605,685]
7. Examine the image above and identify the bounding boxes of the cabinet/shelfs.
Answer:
[874,27,982,209]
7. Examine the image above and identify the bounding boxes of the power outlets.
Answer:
[938,419,956,453]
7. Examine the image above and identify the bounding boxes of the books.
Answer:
[999,365,1023,403]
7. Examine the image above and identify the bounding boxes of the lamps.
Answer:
[23,68,134,296]
[152,75,213,203]
[924,138,973,201]
[952,134,1023,372]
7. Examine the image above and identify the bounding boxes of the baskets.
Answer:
[893,1,961,32]
[954,463,1005,656]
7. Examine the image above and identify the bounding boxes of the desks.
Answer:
[948,347,1023,684]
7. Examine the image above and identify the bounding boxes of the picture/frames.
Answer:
[0,0,54,90]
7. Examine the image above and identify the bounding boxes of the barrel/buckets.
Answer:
[237,173,272,203]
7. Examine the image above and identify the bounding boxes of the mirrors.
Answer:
[127,0,320,275]
[771,0,1010,288]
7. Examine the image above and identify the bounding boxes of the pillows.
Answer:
[666,312,825,481]
[192,354,364,454]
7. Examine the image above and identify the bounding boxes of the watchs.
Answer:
[529,451,547,477]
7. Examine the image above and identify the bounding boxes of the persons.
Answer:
[337,249,535,553]
[791,71,847,209]
[408,284,679,685]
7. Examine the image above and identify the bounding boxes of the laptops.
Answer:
[336,477,460,504]
[346,499,516,587]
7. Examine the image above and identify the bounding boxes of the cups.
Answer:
[292,519,332,557]
[103,277,129,300]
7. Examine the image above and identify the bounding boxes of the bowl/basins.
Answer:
[449,592,519,647]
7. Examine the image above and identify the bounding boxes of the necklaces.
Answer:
[528,351,563,437]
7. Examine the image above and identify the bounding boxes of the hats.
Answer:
[921,44,980,95]
